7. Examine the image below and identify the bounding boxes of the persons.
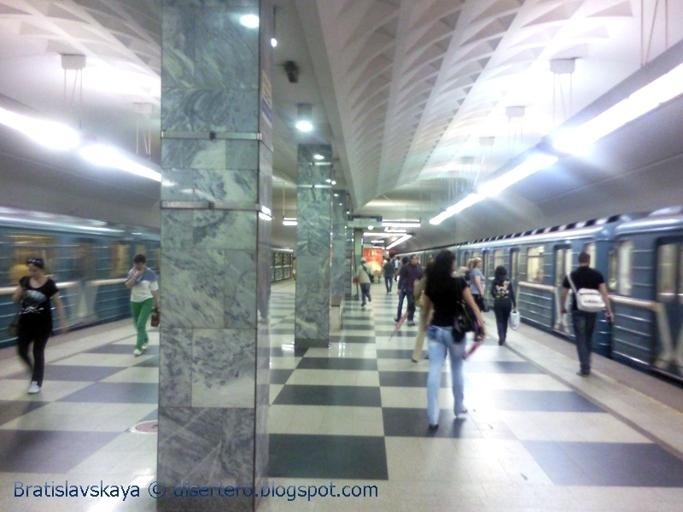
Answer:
[558,250,617,380]
[121,251,161,358]
[8,256,67,397]
[411,251,488,433]
[353,252,518,360]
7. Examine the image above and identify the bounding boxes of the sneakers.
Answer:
[360,293,372,308]
[575,365,593,377]
[394,316,416,328]
[427,406,471,432]
[132,339,148,356]
[27,379,43,395]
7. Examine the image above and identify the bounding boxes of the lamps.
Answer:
[283,61,299,83]
[296,103,314,135]
[386,56,683,249]
[281,181,423,229]
[0,54,162,183]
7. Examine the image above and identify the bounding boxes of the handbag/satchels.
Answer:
[450,281,483,335]
[370,274,375,283]
[573,285,607,313]
[480,296,490,313]
[150,309,159,328]
[7,315,21,337]
[506,309,521,332]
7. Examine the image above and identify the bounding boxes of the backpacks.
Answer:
[491,279,511,305]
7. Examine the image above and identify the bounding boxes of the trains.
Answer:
[395,205,683,382]
[0,206,293,348]
